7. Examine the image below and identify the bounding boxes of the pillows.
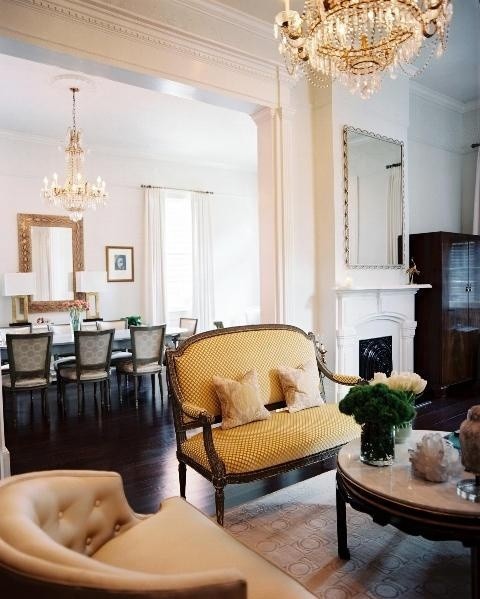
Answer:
[276,358,328,415]
[210,367,273,430]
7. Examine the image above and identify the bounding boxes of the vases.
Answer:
[69,311,82,334]
[394,396,413,443]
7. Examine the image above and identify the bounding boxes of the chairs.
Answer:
[2,332,51,423]
[117,323,165,410]
[0,468,323,599]
[56,328,114,416]
[173,317,200,343]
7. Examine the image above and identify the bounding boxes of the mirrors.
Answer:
[18,213,85,313]
[343,126,408,271]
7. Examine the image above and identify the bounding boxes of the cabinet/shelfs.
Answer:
[409,230,480,392]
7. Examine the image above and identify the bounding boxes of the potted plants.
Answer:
[337,383,418,467]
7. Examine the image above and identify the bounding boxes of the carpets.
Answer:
[209,466,480,599]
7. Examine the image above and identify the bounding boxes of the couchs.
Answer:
[165,324,378,526]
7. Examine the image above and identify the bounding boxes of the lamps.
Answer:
[38,76,112,224]
[75,271,108,320]
[270,0,456,101]
[1,273,36,323]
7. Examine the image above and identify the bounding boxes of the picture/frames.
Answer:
[105,245,135,283]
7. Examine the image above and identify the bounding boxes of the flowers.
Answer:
[62,299,92,328]
[368,370,430,430]
